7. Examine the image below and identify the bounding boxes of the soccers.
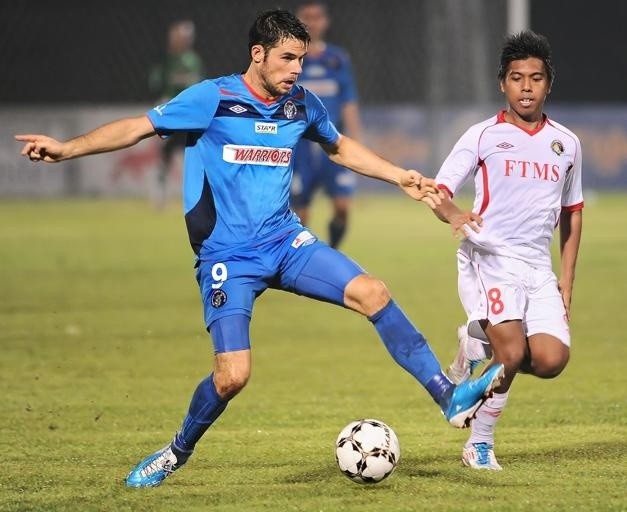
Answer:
[335,419,399,485]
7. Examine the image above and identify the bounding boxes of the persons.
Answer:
[145,17,204,198]
[285,5,362,252]
[426,28,585,471]
[12,8,507,490]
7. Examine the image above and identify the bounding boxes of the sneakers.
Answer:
[445,364,505,428]
[125,432,194,487]
[462,441,502,470]
[446,325,485,385]
